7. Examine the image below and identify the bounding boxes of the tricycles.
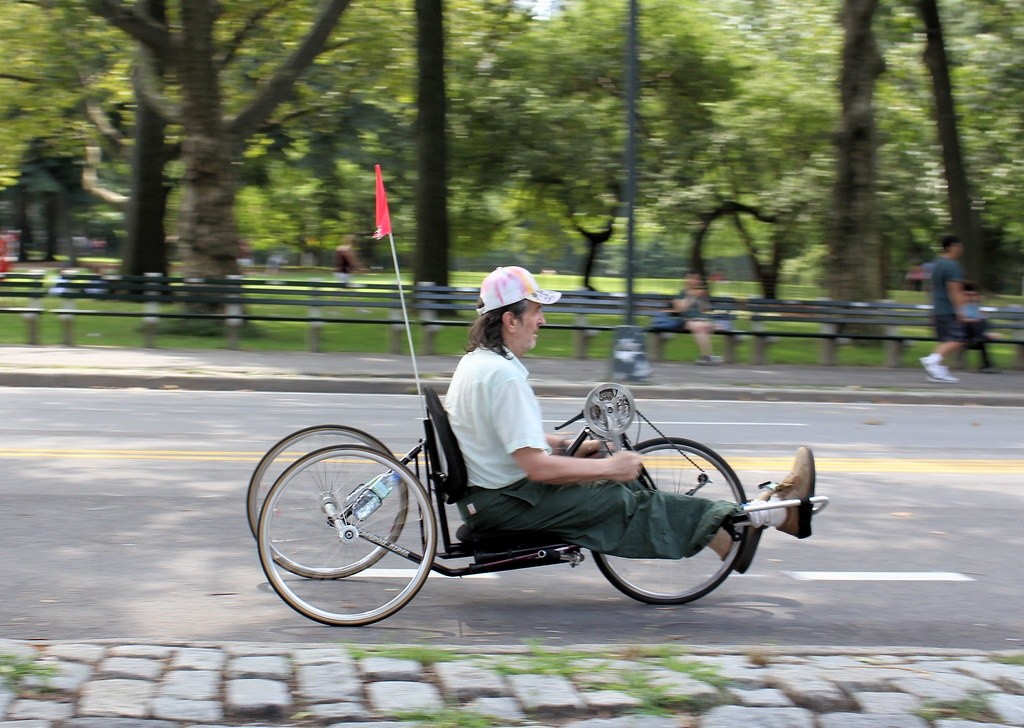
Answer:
[244,381,830,626]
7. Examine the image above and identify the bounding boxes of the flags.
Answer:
[374,166,391,237]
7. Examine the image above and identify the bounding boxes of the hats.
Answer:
[477,266,562,315]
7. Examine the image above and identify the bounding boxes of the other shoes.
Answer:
[921,355,959,384]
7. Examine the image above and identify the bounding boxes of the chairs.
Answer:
[423,386,558,549]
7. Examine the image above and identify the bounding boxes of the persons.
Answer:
[673,270,718,365]
[906,235,1001,383]
[335,234,364,284]
[445,266,816,574]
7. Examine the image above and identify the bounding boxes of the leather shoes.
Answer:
[720,489,771,574]
[773,446,814,539]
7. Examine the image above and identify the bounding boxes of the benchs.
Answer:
[0,267,1024,367]
